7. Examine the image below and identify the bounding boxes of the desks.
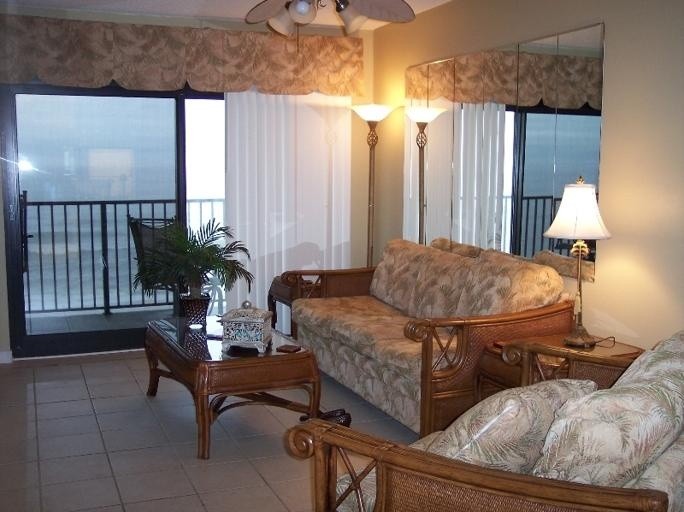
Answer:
[267,277,321,339]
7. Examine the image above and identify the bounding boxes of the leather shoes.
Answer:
[300,409,351,427]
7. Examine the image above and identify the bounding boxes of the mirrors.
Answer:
[404,20,605,284]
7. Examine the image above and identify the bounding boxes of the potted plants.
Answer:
[131,214,256,344]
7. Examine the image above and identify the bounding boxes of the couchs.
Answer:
[281,242,576,400]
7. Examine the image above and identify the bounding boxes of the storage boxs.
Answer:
[216,298,273,355]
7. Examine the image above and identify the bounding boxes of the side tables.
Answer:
[476,330,646,406]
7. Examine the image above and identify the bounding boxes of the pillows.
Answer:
[369,238,475,320]
[456,250,565,317]
[428,237,481,259]
[425,378,598,478]
[536,330,683,487]
[533,251,595,283]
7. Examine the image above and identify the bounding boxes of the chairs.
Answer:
[283,340,684,510]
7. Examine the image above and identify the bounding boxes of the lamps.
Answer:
[403,107,450,244]
[351,103,393,267]
[543,174,615,349]
[245,1,416,38]
